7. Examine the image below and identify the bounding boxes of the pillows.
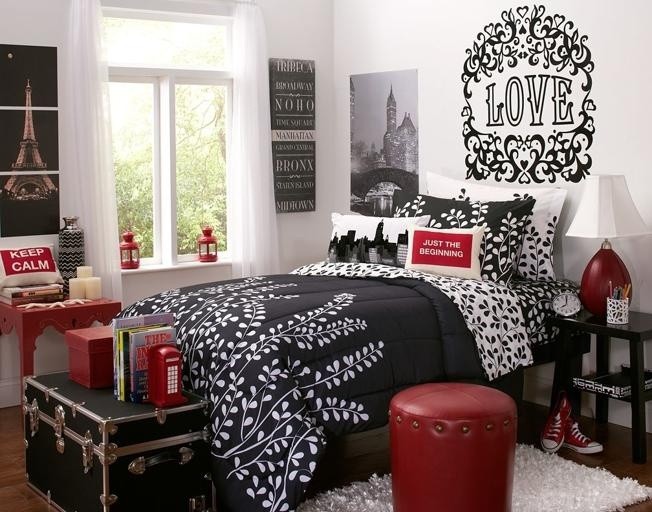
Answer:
[325,168,568,283]
[0,242,62,290]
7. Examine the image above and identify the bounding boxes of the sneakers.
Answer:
[539,397,604,455]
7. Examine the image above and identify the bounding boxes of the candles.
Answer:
[69,264,102,300]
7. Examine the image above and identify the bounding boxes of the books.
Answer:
[0,283,64,306]
[112,313,178,403]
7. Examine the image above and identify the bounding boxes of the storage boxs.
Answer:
[62,327,116,387]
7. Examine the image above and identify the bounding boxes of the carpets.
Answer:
[288,445,651,512]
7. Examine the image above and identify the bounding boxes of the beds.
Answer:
[119,258,583,512]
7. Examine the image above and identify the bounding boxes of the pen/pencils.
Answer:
[608,281,632,300]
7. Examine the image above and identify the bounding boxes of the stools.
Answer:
[389,380,519,512]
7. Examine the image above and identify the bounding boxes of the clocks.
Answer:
[551,292,583,319]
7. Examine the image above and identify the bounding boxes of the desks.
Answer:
[546,307,651,465]
[1,294,120,429]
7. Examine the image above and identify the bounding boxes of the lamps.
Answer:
[564,174,651,317]
